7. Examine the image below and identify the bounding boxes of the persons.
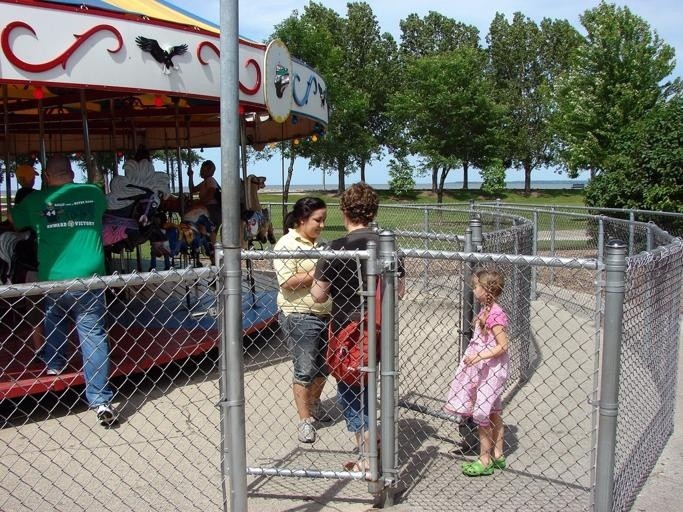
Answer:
[444,270,512,477]
[312,180,406,471]
[8,156,119,426]
[15,163,39,204]
[273,196,332,443]
[187,160,220,210]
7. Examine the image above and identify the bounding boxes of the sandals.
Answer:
[342,437,382,471]
[491,454,506,469]
[460,459,494,476]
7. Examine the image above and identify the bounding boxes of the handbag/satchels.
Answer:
[326,311,381,385]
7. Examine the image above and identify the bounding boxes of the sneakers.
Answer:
[298,422,315,443]
[34,349,46,362]
[308,402,331,421]
[47,364,67,375]
[96,404,118,425]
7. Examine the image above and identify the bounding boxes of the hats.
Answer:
[16,165,39,177]
